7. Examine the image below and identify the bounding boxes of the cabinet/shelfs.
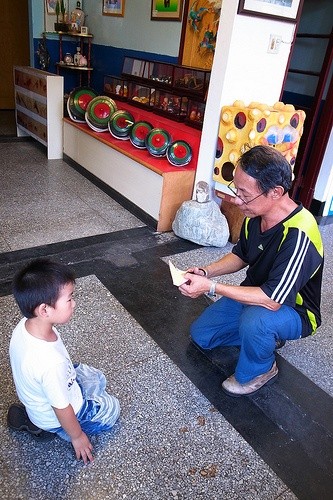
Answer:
[13,65,64,160]
[56,31,94,102]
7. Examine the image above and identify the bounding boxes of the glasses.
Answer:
[226,180,272,206]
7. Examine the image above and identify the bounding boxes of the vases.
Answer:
[71,8,86,33]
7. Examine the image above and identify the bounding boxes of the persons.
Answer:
[7,258,121,465]
[177,146,326,398]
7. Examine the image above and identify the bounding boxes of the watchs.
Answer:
[207,282,217,297]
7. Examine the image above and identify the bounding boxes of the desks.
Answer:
[63,96,223,232]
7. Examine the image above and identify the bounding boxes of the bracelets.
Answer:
[199,268,207,276]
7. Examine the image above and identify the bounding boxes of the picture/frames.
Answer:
[150,0,184,21]
[237,0,304,24]
[47,0,62,15]
[102,0,125,17]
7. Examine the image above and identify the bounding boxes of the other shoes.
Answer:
[275,339,285,349]
[7,403,42,435]
[221,360,280,398]
